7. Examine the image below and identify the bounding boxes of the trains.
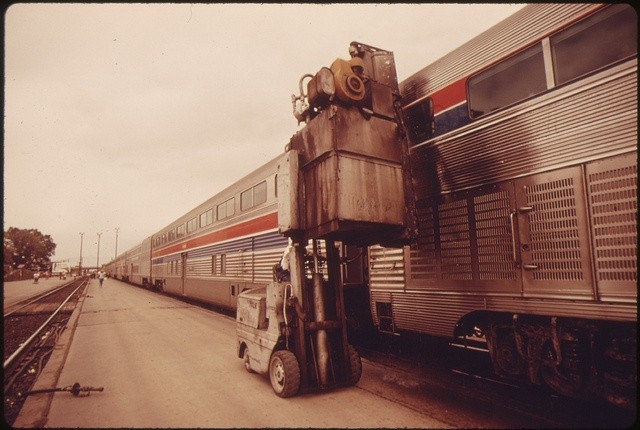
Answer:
[99,2,640,427]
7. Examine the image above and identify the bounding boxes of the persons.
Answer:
[97,269,105,288]
[33,271,39,282]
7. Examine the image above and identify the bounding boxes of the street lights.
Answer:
[79,231,85,268]
[114,225,120,258]
[96,232,101,268]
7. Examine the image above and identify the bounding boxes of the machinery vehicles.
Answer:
[233,42,421,397]
